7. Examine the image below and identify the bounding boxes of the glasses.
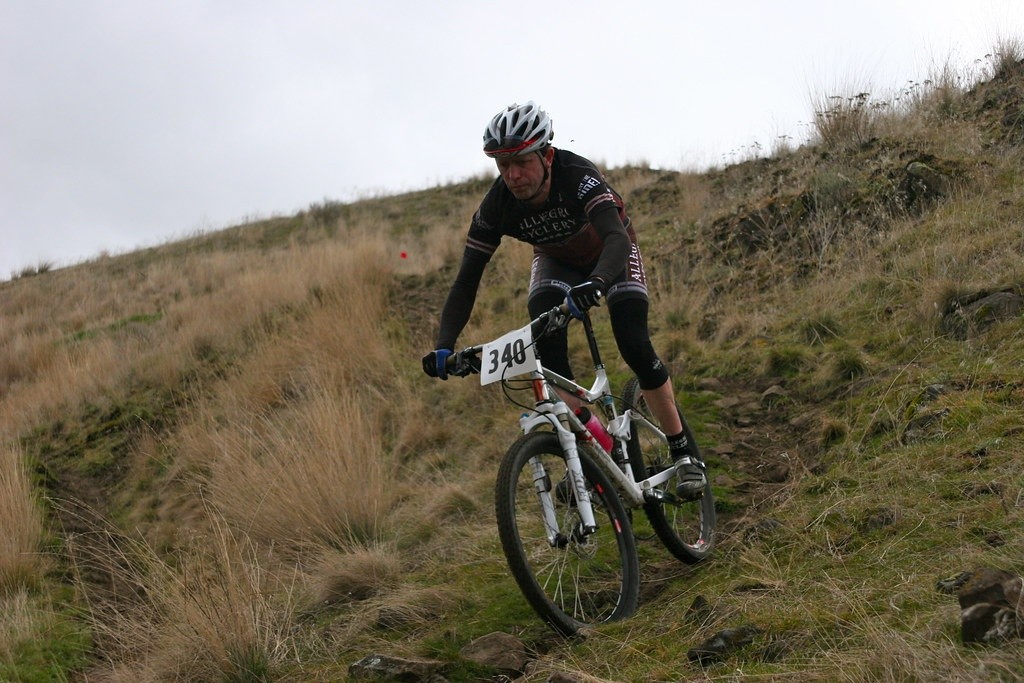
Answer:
[483,135,539,157]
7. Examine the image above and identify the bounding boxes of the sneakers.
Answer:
[673,454,707,495]
[555,467,593,501]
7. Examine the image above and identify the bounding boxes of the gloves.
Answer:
[421,347,453,380]
[566,278,605,320]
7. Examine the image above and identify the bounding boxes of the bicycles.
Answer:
[448,285,720,645]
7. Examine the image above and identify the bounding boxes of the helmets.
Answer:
[484,103,554,158]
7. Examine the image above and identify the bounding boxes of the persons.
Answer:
[422,101,707,497]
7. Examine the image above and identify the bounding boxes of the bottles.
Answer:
[574,406,613,453]
